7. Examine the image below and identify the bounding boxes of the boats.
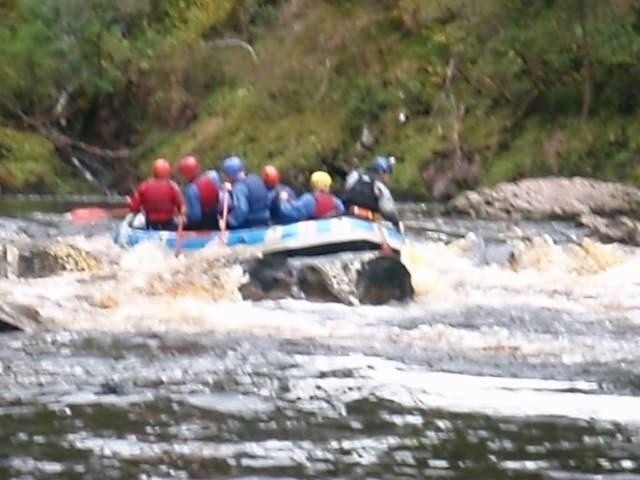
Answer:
[114,206,407,249]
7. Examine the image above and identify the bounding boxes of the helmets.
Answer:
[152,157,171,178]
[259,164,282,190]
[221,154,245,179]
[308,170,333,190]
[367,154,393,178]
[175,154,202,180]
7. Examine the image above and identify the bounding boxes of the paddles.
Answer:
[71,207,130,223]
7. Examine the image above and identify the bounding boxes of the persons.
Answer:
[170,155,234,230]
[278,168,346,226]
[127,154,187,235]
[259,163,299,226]
[220,154,274,229]
[343,154,406,235]
